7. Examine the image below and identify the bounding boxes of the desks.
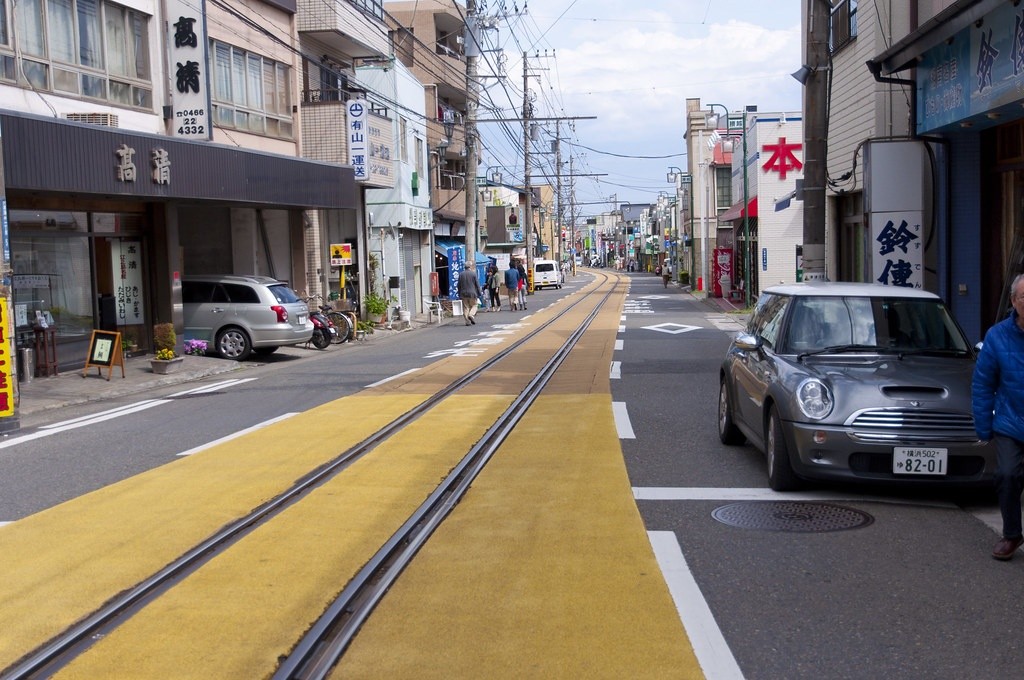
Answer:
[728,290,745,303]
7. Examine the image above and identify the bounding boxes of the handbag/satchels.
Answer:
[518,278,523,290]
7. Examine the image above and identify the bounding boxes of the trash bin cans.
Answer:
[679,270,689,284]
[17,347,34,384]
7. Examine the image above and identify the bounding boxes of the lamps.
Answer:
[779,113,786,126]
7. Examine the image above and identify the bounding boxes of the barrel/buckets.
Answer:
[399,311,410,325]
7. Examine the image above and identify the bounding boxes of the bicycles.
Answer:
[309,293,352,345]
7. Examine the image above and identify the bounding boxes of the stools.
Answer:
[34,325,58,377]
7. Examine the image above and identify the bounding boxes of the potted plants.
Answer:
[122,337,133,358]
[355,253,399,340]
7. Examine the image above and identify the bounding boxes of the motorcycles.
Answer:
[295,289,336,350]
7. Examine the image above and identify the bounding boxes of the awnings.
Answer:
[718,196,757,222]
[435,240,493,267]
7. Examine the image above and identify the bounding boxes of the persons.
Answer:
[487,266,501,312]
[660,262,669,289]
[505,260,529,311]
[458,261,481,325]
[971,275,1023,558]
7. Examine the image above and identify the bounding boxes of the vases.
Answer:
[149,356,184,373]
[130,345,138,353]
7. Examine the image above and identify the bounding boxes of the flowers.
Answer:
[153,323,177,359]
[184,338,208,355]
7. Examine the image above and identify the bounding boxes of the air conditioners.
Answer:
[61,112,119,127]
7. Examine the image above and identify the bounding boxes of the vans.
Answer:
[533,261,562,291]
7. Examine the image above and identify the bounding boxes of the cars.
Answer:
[719,281,1001,506]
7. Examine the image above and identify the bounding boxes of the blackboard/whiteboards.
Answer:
[83,329,124,368]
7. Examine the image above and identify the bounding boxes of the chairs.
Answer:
[423,298,444,324]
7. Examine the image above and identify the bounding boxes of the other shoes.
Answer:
[525,308,527,310]
[468,316,476,324]
[511,308,513,311]
[991,533,1024,558]
[492,307,495,312]
[497,306,501,312]
[466,324,470,325]
[514,303,517,310]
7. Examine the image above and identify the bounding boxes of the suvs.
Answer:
[180,273,315,363]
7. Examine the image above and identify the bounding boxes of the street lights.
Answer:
[474,166,502,253]
[705,104,751,310]
[538,202,555,260]
[656,191,679,284]
[427,141,449,304]
[666,166,695,291]
